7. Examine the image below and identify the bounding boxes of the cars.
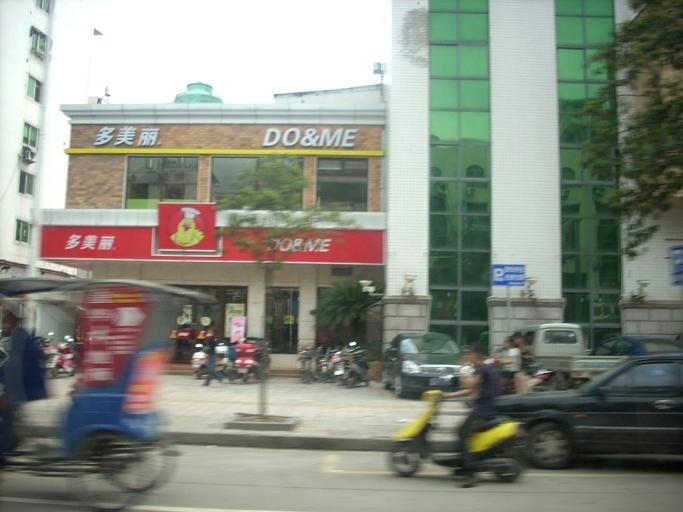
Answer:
[380,331,465,398]
[491,352,683,471]
[590,332,683,356]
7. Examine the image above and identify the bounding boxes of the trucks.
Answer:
[506,323,635,392]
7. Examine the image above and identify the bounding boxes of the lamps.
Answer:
[631,276,652,304]
[517,275,537,303]
[398,275,418,297]
[358,279,385,298]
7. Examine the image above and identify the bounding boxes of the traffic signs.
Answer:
[490,262,525,286]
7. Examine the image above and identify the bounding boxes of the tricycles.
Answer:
[0,277,217,508]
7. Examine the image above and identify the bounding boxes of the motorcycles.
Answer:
[467,353,556,406]
[386,385,526,486]
[188,330,275,385]
[297,340,374,389]
[28,330,77,381]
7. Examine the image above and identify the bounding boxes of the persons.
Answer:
[0,308,50,473]
[202,324,224,386]
[442,347,500,487]
[495,337,523,396]
